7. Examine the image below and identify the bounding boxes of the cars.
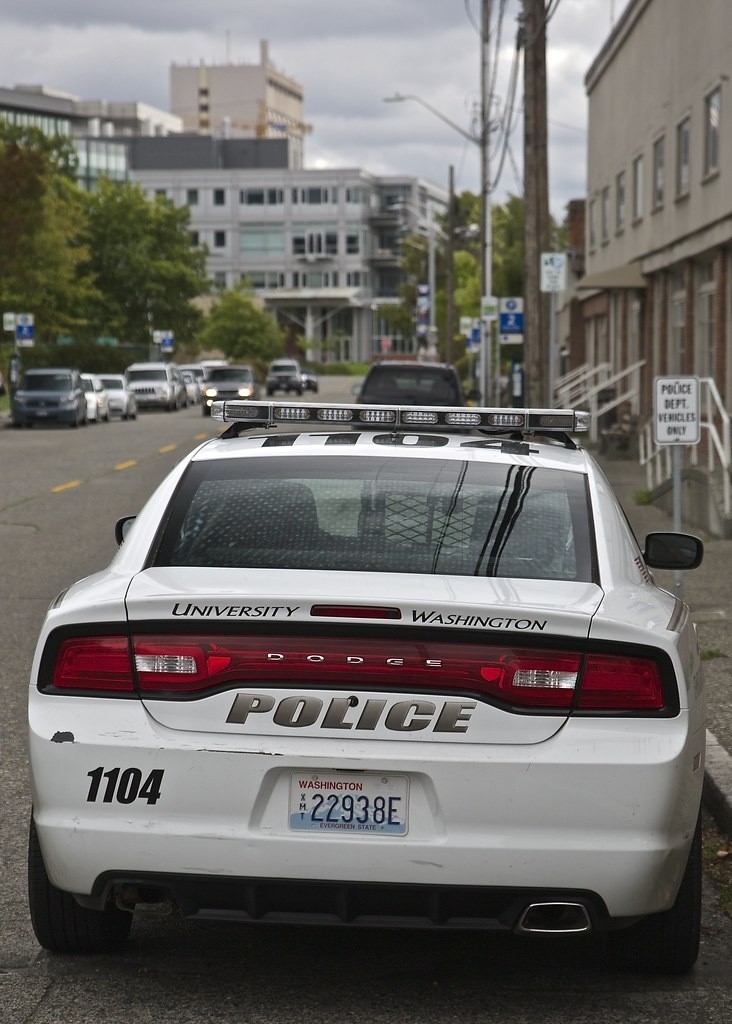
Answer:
[298,371,318,394]
[265,359,305,396]
[349,359,483,426]
[183,369,203,406]
[23,398,709,983]
[98,375,139,421]
[201,365,260,414]
[77,374,111,423]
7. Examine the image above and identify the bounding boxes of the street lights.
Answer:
[385,198,437,331]
[381,93,491,299]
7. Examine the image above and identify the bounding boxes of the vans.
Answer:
[123,362,188,411]
[11,368,88,428]
